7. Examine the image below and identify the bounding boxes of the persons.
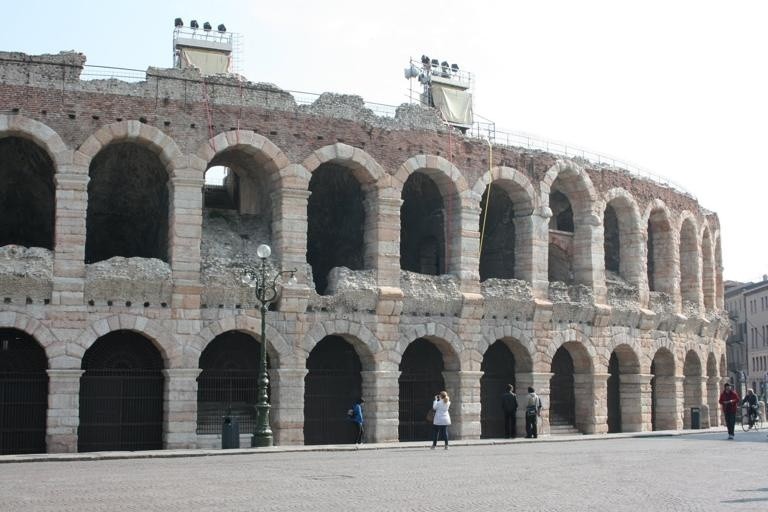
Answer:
[350,397,367,445]
[531,396,542,436]
[523,385,539,439]
[717,383,740,439]
[430,390,453,450]
[500,384,519,439]
[741,389,760,430]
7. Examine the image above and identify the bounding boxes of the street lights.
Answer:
[241,243,298,449]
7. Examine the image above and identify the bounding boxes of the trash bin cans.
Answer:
[222,415,240,449]
[690,407,700,429]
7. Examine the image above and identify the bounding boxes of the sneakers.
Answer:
[431,446,434,449]
[445,445,448,449]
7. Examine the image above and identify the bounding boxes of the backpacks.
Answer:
[347,409,354,417]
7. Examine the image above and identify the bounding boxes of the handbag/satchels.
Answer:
[427,409,437,422]
[527,406,538,415]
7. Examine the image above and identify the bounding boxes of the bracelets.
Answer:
[730,399,733,403]
